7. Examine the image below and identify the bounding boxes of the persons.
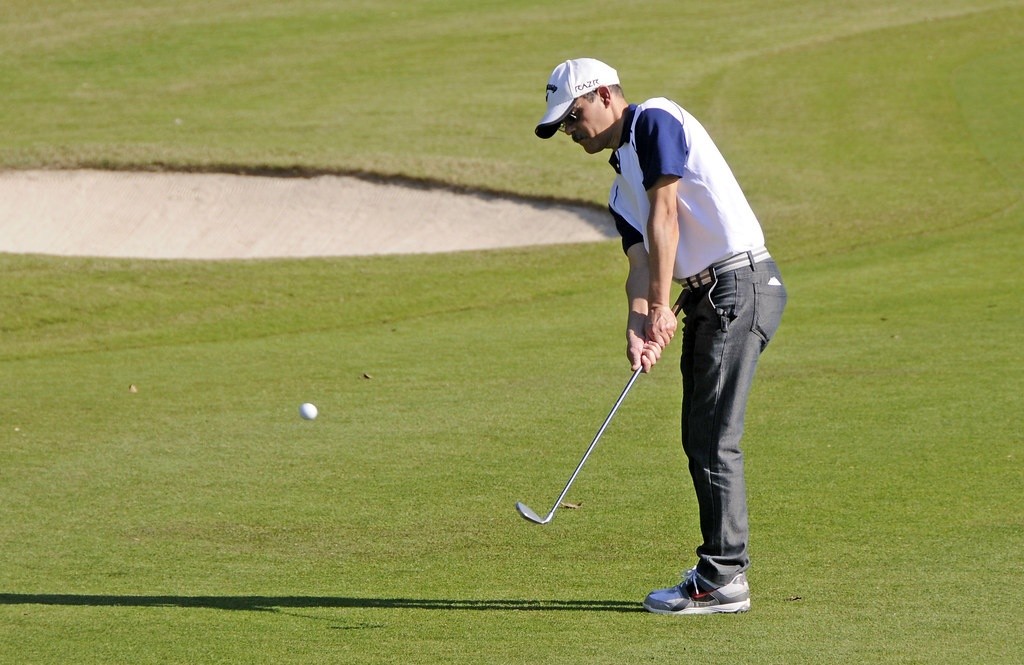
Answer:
[534,58,787,615]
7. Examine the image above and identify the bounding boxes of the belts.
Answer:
[680,245,772,291]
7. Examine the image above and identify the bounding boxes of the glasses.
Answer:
[555,94,603,134]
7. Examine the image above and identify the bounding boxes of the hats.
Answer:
[533,57,622,140]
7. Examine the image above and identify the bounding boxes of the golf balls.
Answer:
[299,403,318,421]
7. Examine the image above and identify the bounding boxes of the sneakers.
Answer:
[642,563,752,616]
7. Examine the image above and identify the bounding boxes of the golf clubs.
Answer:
[514,288,692,528]
